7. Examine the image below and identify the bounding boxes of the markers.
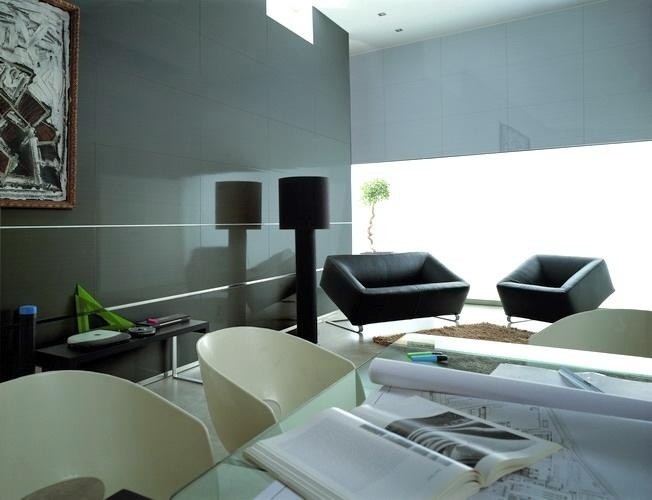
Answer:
[407,352,448,362]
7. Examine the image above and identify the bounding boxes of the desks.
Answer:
[35,319,210,383]
[165,332,652,499]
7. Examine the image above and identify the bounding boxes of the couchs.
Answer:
[319,251,469,334]
[497,251,615,323]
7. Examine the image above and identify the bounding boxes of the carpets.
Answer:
[373,322,536,376]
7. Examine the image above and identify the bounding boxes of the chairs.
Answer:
[526,308,652,357]
[196,327,367,463]
[0,370,219,500]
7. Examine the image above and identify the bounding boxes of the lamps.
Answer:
[279,176,330,344]
[215,181,262,326]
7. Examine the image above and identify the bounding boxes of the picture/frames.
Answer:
[0,0,81,210]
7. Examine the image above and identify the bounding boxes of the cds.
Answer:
[128,326,156,338]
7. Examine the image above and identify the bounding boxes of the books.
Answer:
[244,394,563,500]
[252,354,652,500]
